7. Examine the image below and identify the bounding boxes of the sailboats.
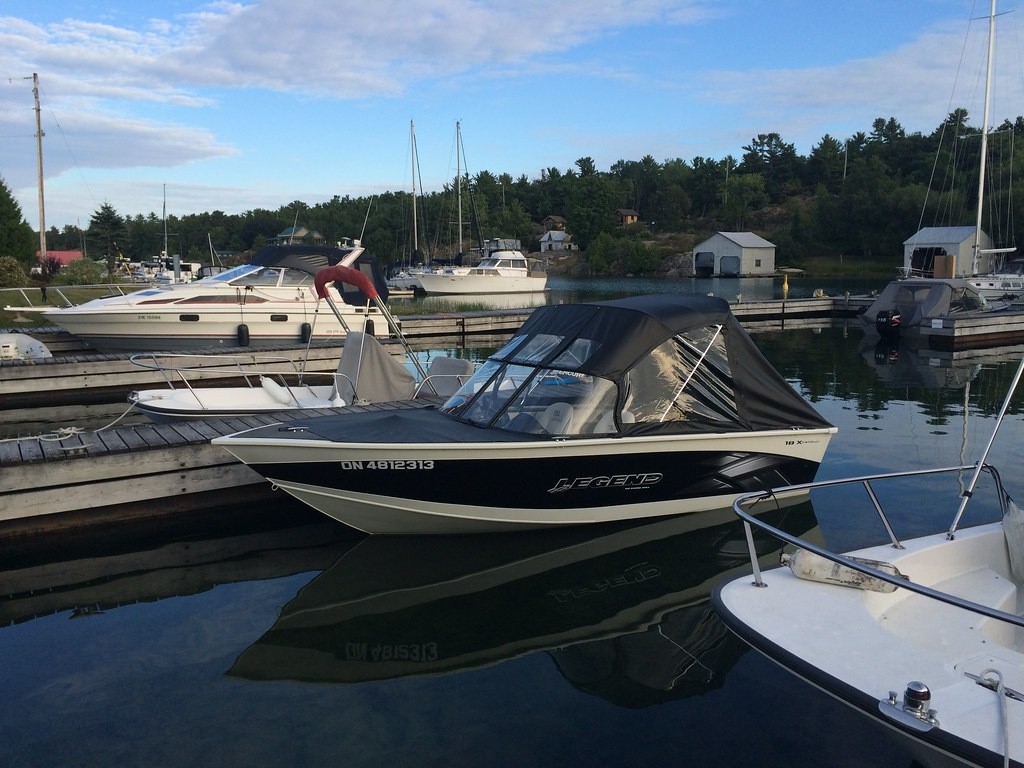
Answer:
[856,347,1024,504]
[401,122,550,294]
[387,120,470,289]
[864,0,1024,346]
[417,292,547,311]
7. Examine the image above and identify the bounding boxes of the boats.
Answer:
[713,349,1024,768]
[230,491,832,707]
[209,294,833,531]
[3,238,405,350]
[123,339,485,426]
